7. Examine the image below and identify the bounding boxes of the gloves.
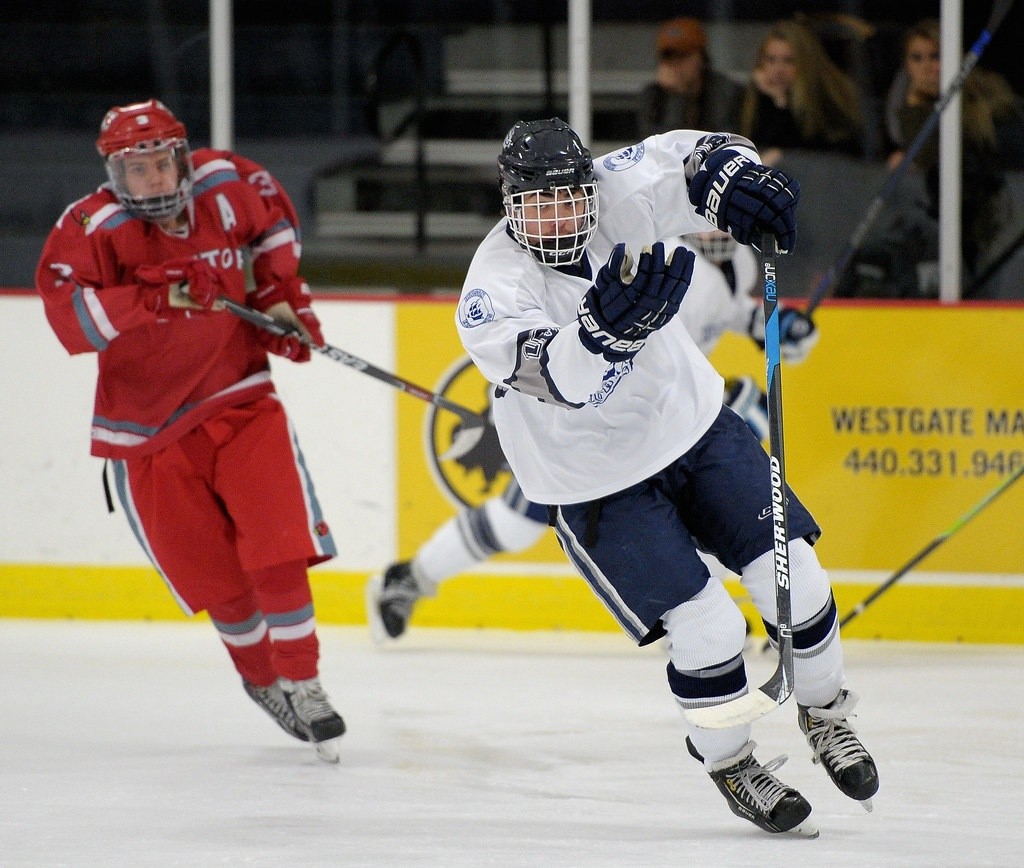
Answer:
[749,302,818,365]
[688,148,800,257]
[733,380,768,442]
[245,277,324,363]
[577,242,696,358]
[135,258,236,314]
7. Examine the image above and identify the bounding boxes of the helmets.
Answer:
[97,100,193,226]
[498,118,600,267]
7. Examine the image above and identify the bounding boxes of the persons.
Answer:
[35,99,345,763]
[634,15,1023,299]
[455,117,879,837]
[369,230,816,642]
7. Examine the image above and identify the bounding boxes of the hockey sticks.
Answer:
[801,0,1016,317]
[176,279,486,462]
[682,249,797,732]
[762,466,1024,666]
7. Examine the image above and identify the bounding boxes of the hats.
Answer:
[649,19,709,58]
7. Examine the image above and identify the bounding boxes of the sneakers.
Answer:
[685,735,819,839]
[797,689,880,812]
[281,675,346,765]
[365,560,421,646]
[245,680,310,743]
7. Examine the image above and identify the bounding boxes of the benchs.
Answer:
[307,63,655,257]
